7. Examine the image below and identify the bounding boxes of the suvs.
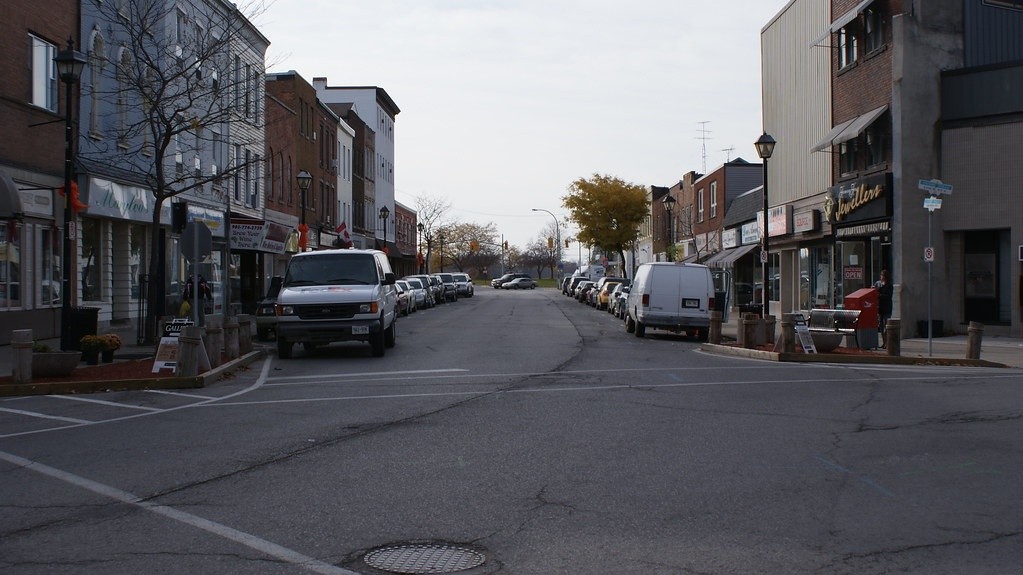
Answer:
[274,245,399,360]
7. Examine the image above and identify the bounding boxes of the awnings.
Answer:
[704,248,735,268]
[683,251,712,263]
[810,117,858,154]
[377,238,401,257]
[0,170,24,220]
[833,103,888,146]
[832,0,873,33]
[717,244,757,268]
[809,7,854,47]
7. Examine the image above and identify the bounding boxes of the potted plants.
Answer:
[29,340,84,379]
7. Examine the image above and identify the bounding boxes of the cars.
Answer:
[254,296,276,342]
[561,274,633,323]
[502,277,536,290]
[393,271,475,317]
[490,273,521,289]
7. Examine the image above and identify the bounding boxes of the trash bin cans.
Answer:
[71,307,101,351]
[715,291,726,319]
[737,304,763,318]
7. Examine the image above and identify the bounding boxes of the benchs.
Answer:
[795,309,863,350]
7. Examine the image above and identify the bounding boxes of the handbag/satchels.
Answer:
[179,301,190,318]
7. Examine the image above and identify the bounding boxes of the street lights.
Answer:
[661,193,676,262]
[379,206,391,253]
[417,222,425,274]
[754,132,777,318]
[295,167,312,253]
[51,33,88,354]
[532,208,560,281]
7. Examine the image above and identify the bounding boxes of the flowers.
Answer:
[80,334,122,353]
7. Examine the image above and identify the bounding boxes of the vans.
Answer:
[624,260,716,343]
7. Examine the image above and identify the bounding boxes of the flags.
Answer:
[336,223,352,247]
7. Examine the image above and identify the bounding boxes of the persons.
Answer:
[183,274,212,327]
[870,270,893,348]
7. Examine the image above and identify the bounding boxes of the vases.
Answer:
[85,350,99,365]
[102,349,114,363]
[795,331,846,352]
